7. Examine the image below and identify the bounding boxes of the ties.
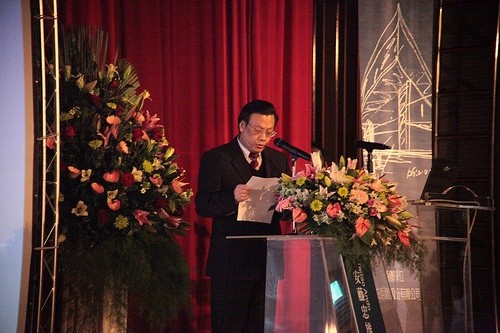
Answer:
[249,152,259,167]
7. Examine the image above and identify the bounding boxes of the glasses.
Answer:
[244,123,276,138]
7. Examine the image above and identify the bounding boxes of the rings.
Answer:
[240,194,244,197]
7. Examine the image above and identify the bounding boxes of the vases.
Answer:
[60,275,128,333]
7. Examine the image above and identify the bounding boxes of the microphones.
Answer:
[354,140,391,150]
[274,138,311,160]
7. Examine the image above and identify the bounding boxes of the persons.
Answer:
[195,100,294,333]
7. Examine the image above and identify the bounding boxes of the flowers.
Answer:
[43,58,193,293]
[270,151,430,281]
